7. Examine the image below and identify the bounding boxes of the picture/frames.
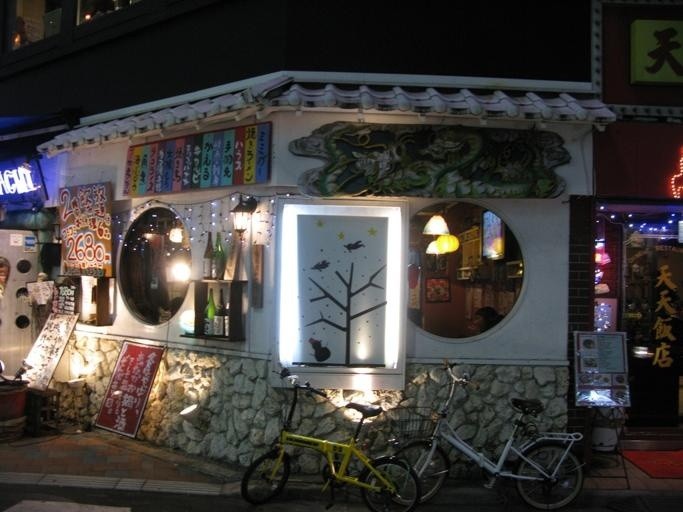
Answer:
[425,277,452,304]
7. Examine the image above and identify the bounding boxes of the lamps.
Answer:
[230,195,258,248]
[422,213,460,256]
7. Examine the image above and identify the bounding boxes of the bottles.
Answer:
[203,233,224,281]
[204,288,228,337]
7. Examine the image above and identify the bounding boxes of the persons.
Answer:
[468,306,504,337]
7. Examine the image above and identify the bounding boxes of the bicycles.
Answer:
[240,366,421,512]
[381,361,591,512]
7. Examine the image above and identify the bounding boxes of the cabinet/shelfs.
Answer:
[179,278,249,343]
[55,272,117,327]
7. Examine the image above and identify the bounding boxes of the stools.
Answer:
[26,389,61,437]
